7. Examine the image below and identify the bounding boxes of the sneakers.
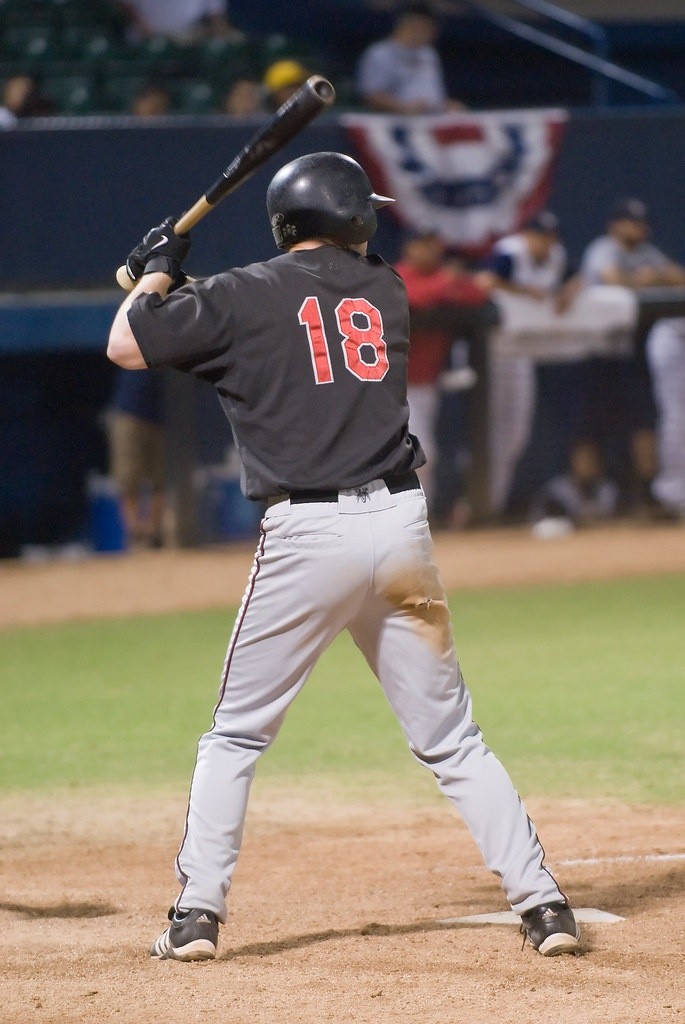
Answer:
[519,903,583,957]
[149,906,219,961]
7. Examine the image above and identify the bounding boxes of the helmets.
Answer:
[267,151,395,248]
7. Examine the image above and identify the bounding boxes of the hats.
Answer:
[614,201,649,222]
[260,61,313,99]
[525,211,564,237]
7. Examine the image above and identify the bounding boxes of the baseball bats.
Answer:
[112,72,341,296]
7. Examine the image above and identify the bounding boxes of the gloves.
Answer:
[126,242,187,293]
[142,216,190,281]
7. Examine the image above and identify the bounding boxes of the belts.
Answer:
[290,471,421,506]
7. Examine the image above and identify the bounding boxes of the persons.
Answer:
[0,0,685,556]
[105,152,584,958]
